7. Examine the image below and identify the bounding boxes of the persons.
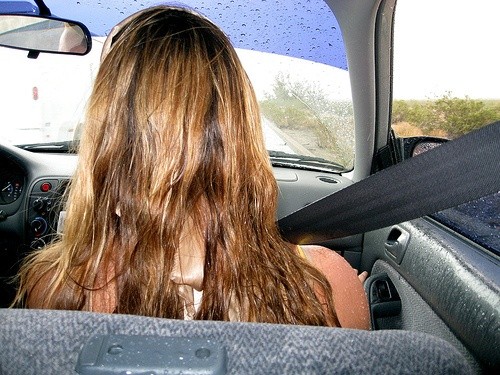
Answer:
[22,5,372,332]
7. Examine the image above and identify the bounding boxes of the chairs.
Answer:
[0,308,473,375]
